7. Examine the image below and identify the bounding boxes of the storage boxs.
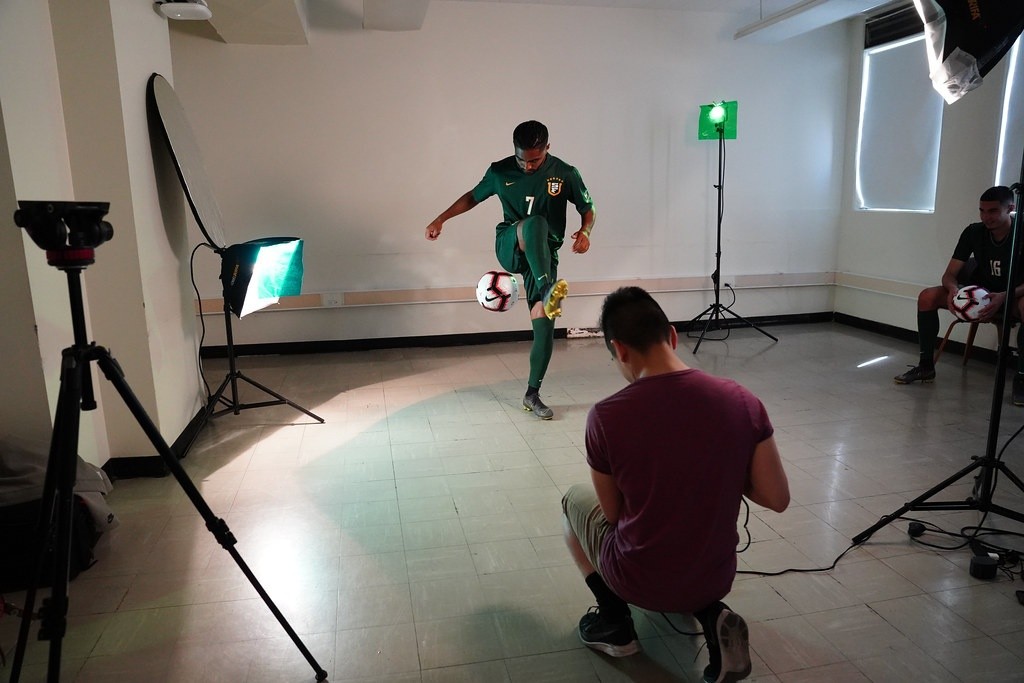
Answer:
[0,491,97,596]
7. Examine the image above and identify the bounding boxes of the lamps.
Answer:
[219,237,301,319]
[160,0,212,20]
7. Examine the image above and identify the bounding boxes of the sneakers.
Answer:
[543,279,568,320]
[579,612,642,657]
[702,601,752,683]
[894,365,937,384]
[523,392,554,420]
[1013,372,1024,405]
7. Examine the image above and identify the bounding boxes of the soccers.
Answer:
[476,271,520,313]
[953,286,990,324]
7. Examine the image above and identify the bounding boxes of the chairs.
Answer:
[931,313,1021,389]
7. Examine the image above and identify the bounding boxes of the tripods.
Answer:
[6,201,328,683]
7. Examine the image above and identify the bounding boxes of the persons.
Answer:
[894,186,1024,407]
[560,286,791,683]
[425,120,595,420]
